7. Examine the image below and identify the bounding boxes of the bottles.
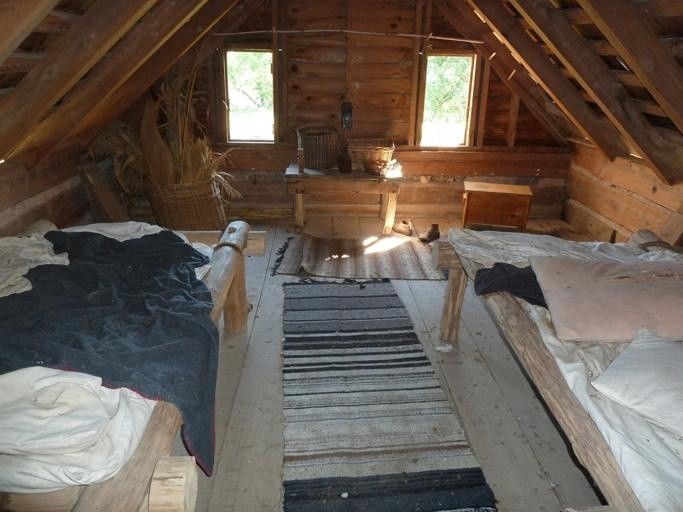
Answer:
[336,140,351,174]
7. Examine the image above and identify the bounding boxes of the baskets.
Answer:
[297,128,337,170]
[146,178,228,233]
[344,138,396,171]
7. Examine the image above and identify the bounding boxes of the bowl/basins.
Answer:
[363,158,392,177]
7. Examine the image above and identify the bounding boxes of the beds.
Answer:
[0,217,252,510]
[426,226,681,510]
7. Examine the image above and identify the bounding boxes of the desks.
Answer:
[283,158,403,238]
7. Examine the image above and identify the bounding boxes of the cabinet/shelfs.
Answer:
[459,180,534,230]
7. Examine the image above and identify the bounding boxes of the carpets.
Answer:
[279,277,498,510]
[267,234,451,284]
